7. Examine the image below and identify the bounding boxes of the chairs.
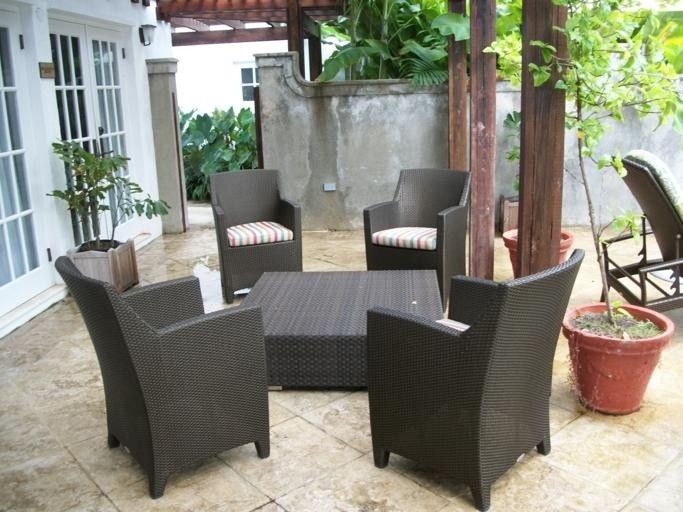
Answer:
[599,150,683,314]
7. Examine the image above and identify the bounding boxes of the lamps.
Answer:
[138,24,157,47]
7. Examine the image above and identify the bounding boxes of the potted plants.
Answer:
[482,0,683,414]
[45,139,172,292]
